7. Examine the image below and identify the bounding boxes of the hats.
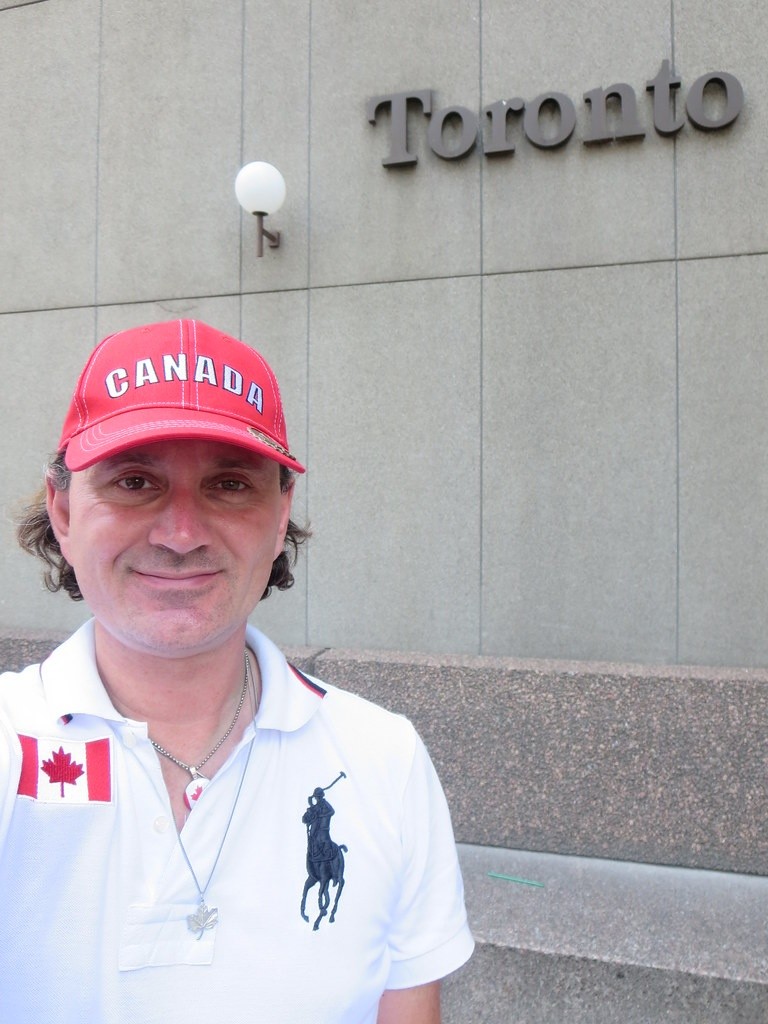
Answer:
[58,318,305,474]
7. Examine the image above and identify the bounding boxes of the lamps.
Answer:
[235,162,286,257]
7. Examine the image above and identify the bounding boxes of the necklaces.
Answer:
[120,655,259,941]
[150,646,248,811]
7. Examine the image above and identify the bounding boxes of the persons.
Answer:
[2,319,476,1024]
